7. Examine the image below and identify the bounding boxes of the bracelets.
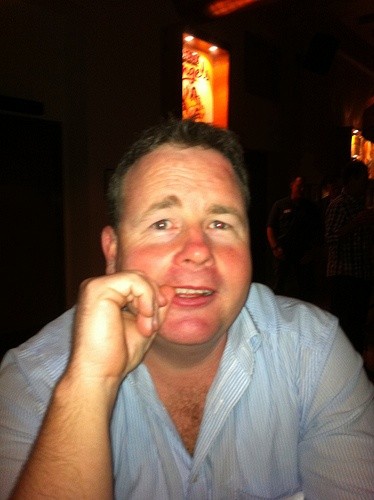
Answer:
[271,245,279,251]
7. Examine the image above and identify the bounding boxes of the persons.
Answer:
[261,168,324,308]
[1,107,374,499]
[322,159,371,377]
